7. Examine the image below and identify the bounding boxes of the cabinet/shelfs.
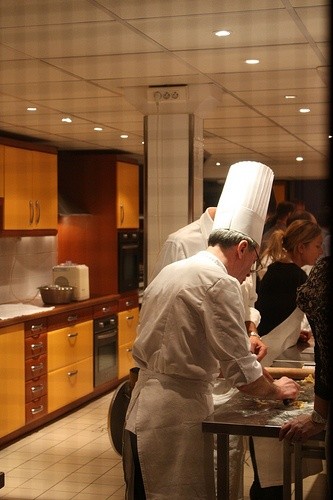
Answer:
[0,137,59,238]
[0,294,141,451]
[58,149,138,299]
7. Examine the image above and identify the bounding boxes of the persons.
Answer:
[149,206,267,500]
[256,189,317,280]
[124,160,301,500]
[279,256,333,500]
[248,219,323,500]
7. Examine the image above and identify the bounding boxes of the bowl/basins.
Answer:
[37,284,75,305]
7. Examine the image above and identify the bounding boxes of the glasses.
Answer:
[248,242,265,275]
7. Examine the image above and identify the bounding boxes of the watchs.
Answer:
[312,411,327,423]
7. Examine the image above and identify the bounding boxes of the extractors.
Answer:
[58,205,94,218]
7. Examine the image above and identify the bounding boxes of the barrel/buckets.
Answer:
[52,260,90,301]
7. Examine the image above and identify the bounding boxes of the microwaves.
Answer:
[118,228,141,293]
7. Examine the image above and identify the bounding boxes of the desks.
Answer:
[201,336,333,500]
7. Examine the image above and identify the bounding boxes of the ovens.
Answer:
[93,303,119,398]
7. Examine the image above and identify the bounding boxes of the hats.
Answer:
[214,159,276,246]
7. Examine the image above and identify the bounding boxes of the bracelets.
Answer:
[249,332,262,341]
